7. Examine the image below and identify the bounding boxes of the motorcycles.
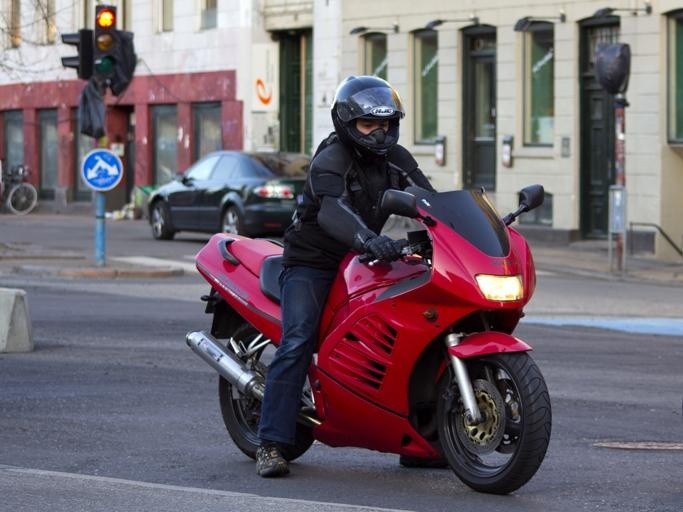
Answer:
[186,186,552,495]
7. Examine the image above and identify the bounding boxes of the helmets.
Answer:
[331,74,405,165]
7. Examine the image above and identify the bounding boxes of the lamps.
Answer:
[515,14,566,32]
[424,17,478,29]
[594,5,652,20]
[351,26,398,33]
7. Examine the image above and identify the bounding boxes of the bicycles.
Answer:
[1,167,38,215]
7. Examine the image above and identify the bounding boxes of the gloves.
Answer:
[363,234,400,263]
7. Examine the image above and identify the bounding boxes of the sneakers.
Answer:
[256,443,290,478]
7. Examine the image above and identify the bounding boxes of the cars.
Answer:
[146,150,312,239]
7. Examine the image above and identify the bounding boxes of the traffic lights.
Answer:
[93,4,119,80]
[60,29,92,80]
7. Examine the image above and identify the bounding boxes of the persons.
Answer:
[256,72,442,479]
